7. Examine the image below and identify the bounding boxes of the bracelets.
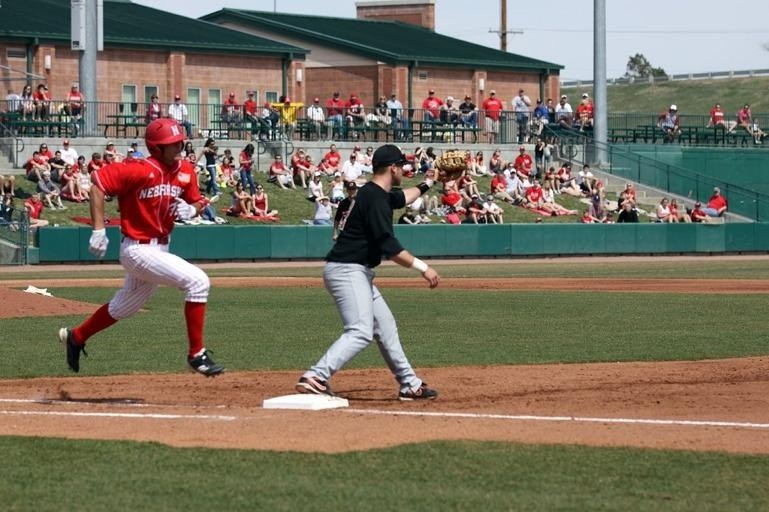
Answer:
[409,255,429,273]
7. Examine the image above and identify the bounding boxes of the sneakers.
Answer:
[59,328,89,373]
[399,383,437,401]
[296,376,337,396]
[187,350,226,378]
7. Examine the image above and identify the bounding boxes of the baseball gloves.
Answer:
[434,151,468,184]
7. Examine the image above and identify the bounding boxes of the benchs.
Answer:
[521,122,768,149]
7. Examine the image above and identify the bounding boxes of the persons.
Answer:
[58,119,225,375]
[648,186,728,224]
[1,85,646,227]
[294,144,442,400]
[657,102,767,145]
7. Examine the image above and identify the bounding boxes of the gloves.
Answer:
[175,198,197,222]
[89,228,109,258]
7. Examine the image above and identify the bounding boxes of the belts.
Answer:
[120,236,168,245]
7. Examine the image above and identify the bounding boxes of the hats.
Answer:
[348,182,356,190]
[373,145,413,167]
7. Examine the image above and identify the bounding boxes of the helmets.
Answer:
[145,120,186,158]
